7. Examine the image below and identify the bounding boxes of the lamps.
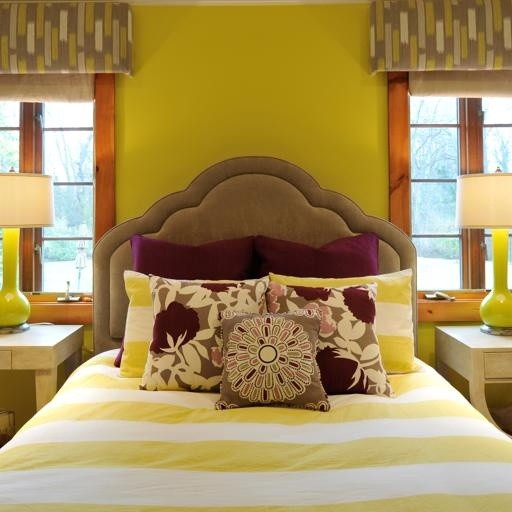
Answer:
[0,167,54,334]
[456,166,511,335]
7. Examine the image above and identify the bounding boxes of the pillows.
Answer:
[114,233,255,369]
[269,267,415,374]
[258,233,381,279]
[214,307,330,412]
[267,281,398,399]
[138,272,269,393]
[118,268,156,380]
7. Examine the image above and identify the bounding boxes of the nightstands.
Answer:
[1,325,84,413]
[436,326,511,438]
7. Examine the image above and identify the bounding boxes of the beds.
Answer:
[0,157,511,511]
